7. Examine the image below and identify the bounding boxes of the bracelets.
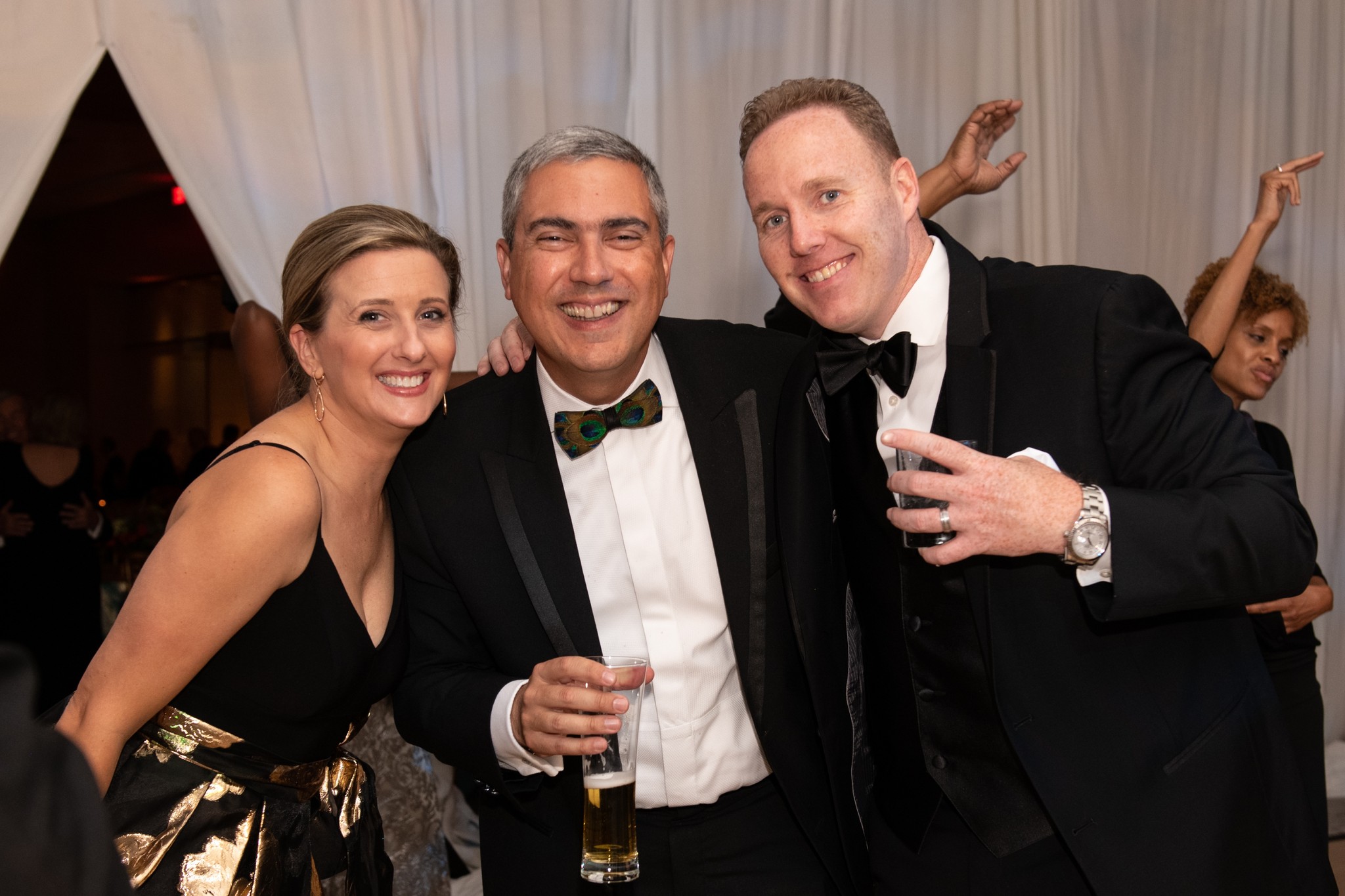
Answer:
[1062,481,1113,570]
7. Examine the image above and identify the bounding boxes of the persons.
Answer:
[738,76,1322,896]
[390,125,866,895]
[44,203,533,896]
[1,395,250,721]
[764,101,1340,895]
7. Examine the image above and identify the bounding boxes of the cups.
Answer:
[897,439,978,548]
[575,655,647,883]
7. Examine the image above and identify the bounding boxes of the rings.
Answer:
[940,510,952,534]
[1274,163,1283,174]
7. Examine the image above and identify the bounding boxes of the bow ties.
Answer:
[815,331,917,399]
[553,379,664,461]
